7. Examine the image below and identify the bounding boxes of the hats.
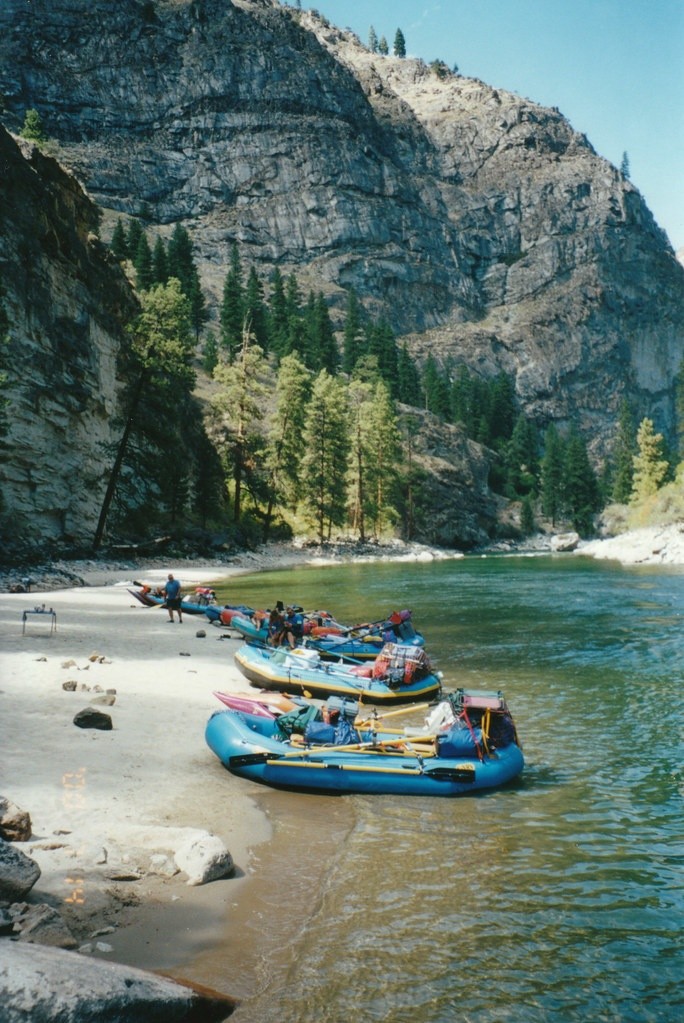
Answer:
[276,601,284,610]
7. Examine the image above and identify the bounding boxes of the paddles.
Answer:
[266,759,475,784]
[320,617,410,654]
[294,641,364,666]
[150,601,167,610]
[229,733,449,768]
[245,636,357,677]
[355,701,439,725]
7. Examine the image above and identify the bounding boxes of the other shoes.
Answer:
[166,620,174,623]
[180,620,183,624]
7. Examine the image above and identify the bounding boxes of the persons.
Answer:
[252,609,270,631]
[269,610,286,647]
[164,574,182,623]
[284,607,304,650]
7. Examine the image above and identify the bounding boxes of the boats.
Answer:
[233,643,444,704]
[205,603,255,621]
[205,688,525,797]
[126,588,155,606]
[138,587,217,613]
[230,608,425,658]
[213,691,365,729]
[221,608,270,625]
